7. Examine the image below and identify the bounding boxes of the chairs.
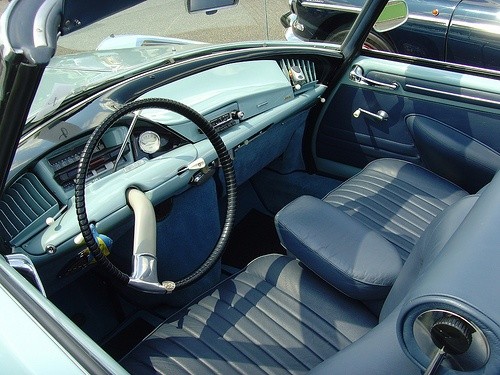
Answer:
[117,171,500,375]
[275,111,500,299]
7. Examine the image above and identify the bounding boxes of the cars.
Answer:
[280,0,500,71]
